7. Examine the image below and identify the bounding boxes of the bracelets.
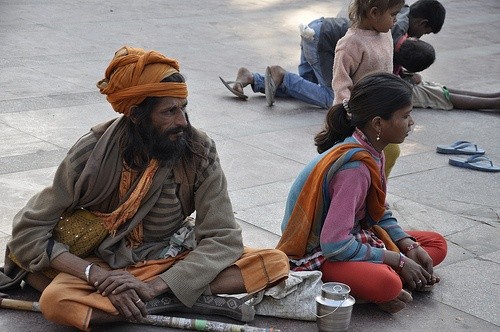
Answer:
[395,253,405,275]
[403,242,419,254]
[85,263,101,282]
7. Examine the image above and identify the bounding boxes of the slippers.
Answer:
[219,75,248,99]
[449,155,500,172]
[265,66,277,107]
[437,140,485,156]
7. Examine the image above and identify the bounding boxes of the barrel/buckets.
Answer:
[308,282,356,332]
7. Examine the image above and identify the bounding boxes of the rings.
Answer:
[417,280,421,283]
[136,299,141,303]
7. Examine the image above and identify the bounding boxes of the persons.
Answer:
[275,72,447,315]
[332,0,404,179]
[0,46,289,332]
[393,0,500,110]
[218,17,435,109]
